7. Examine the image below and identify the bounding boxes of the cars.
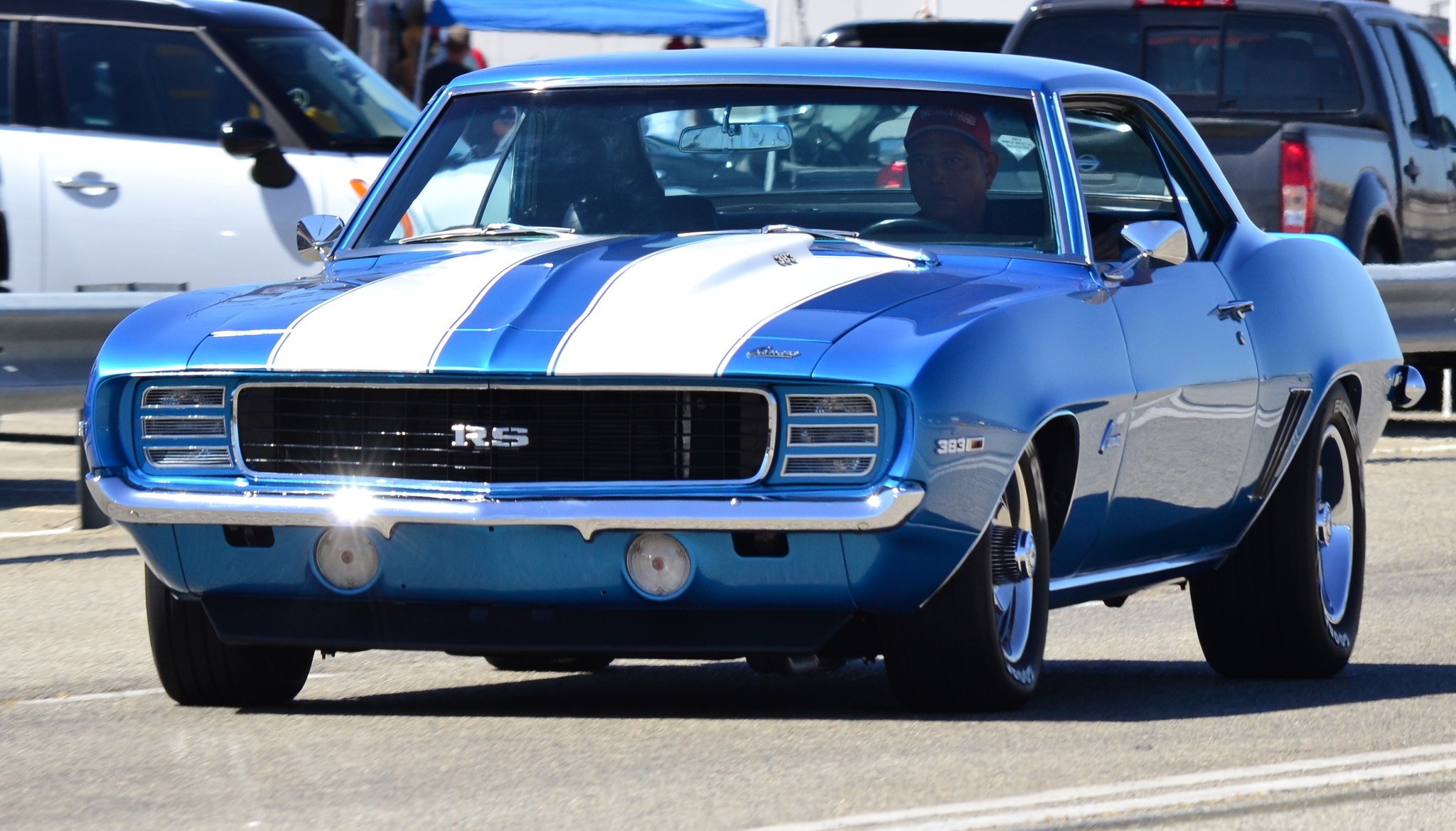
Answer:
[82,47,1427,716]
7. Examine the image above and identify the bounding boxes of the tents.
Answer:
[410,1,771,114]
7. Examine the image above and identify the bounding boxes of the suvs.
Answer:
[643,0,1456,266]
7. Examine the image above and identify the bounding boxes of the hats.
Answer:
[903,103,991,153]
[439,23,472,50]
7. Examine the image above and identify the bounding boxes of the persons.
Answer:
[665,36,704,51]
[898,102,1120,265]
[402,22,487,108]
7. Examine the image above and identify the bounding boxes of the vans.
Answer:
[0,0,476,311]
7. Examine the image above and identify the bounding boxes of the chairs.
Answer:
[558,117,717,231]
